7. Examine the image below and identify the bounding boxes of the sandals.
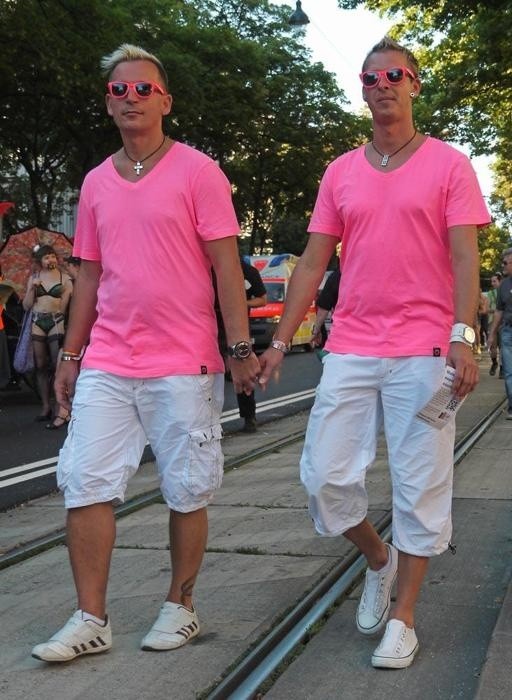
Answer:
[46,415,72,430]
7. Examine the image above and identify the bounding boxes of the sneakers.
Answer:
[32,608,113,663]
[505,404,512,420]
[224,371,232,382]
[140,599,201,652]
[355,542,400,635]
[489,362,504,379]
[244,414,258,433]
[371,619,420,668]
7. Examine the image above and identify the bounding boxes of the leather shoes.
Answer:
[36,408,53,423]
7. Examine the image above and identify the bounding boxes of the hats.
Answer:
[63,254,82,266]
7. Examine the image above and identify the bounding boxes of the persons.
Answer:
[32,44,264,665]
[309,268,341,348]
[472,249,512,420]
[44,256,89,430]
[244,37,494,672]
[22,244,71,422]
[211,259,268,435]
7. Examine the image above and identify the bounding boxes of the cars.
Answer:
[242,277,323,352]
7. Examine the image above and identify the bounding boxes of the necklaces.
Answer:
[372,129,417,167]
[124,134,166,176]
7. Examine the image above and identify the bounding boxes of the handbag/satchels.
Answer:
[14,309,36,374]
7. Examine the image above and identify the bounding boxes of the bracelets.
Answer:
[59,351,81,361]
[311,331,320,335]
[269,341,289,355]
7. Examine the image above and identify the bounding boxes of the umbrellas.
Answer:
[0,225,73,303]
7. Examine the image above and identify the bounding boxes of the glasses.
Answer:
[359,65,416,90]
[108,79,165,99]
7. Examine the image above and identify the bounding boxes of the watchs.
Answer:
[229,340,253,360]
[449,322,476,349]
